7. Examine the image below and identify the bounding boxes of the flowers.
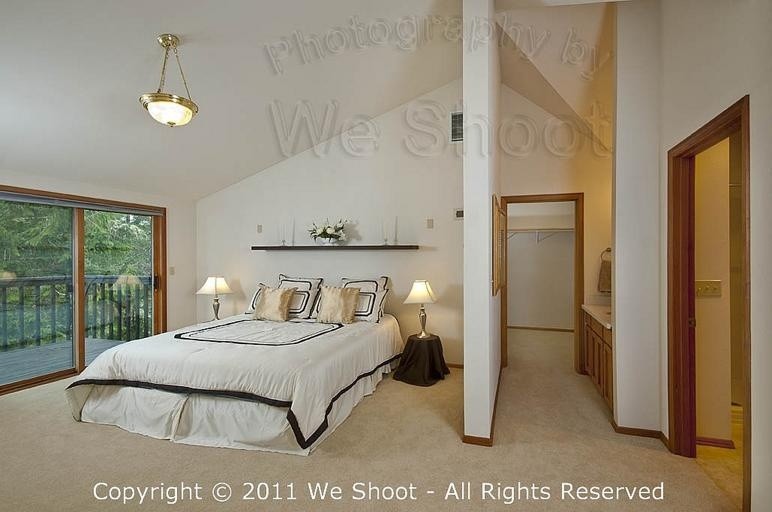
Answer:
[306,218,353,241]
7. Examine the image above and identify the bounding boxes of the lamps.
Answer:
[108,274,145,310]
[195,276,234,321]
[403,279,437,338]
[138,35,198,129]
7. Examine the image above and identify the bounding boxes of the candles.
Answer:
[383,221,387,241]
[281,223,285,240]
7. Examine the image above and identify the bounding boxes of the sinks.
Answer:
[599,309,611,318]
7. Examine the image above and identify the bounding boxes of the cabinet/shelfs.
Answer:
[579,308,614,414]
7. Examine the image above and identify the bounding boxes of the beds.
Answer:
[102,312,397,452]
[393,334,451,387]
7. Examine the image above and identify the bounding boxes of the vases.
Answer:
[320,236,341,246]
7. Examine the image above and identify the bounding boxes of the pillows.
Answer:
[315,284,361,324]
[341,276,388,317]
[310,288,390,324]
[276,273,323,291]
[245,283,321,319]
[252,285,298,322]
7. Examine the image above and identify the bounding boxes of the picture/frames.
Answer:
[492,195,507,294]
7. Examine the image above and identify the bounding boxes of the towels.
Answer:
[598,259,612,294]
